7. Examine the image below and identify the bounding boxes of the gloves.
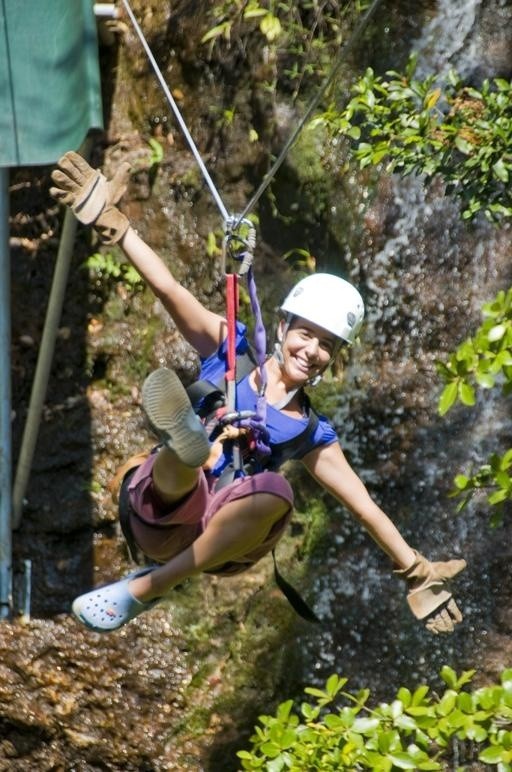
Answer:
[50,150,132,247]
[391,549,468,640]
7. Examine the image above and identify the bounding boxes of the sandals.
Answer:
[142,366,212,469]
[70,565,168,635]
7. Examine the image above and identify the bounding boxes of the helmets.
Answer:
[280,273,365,344]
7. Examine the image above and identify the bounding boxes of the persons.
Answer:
[48,151,467,636]
